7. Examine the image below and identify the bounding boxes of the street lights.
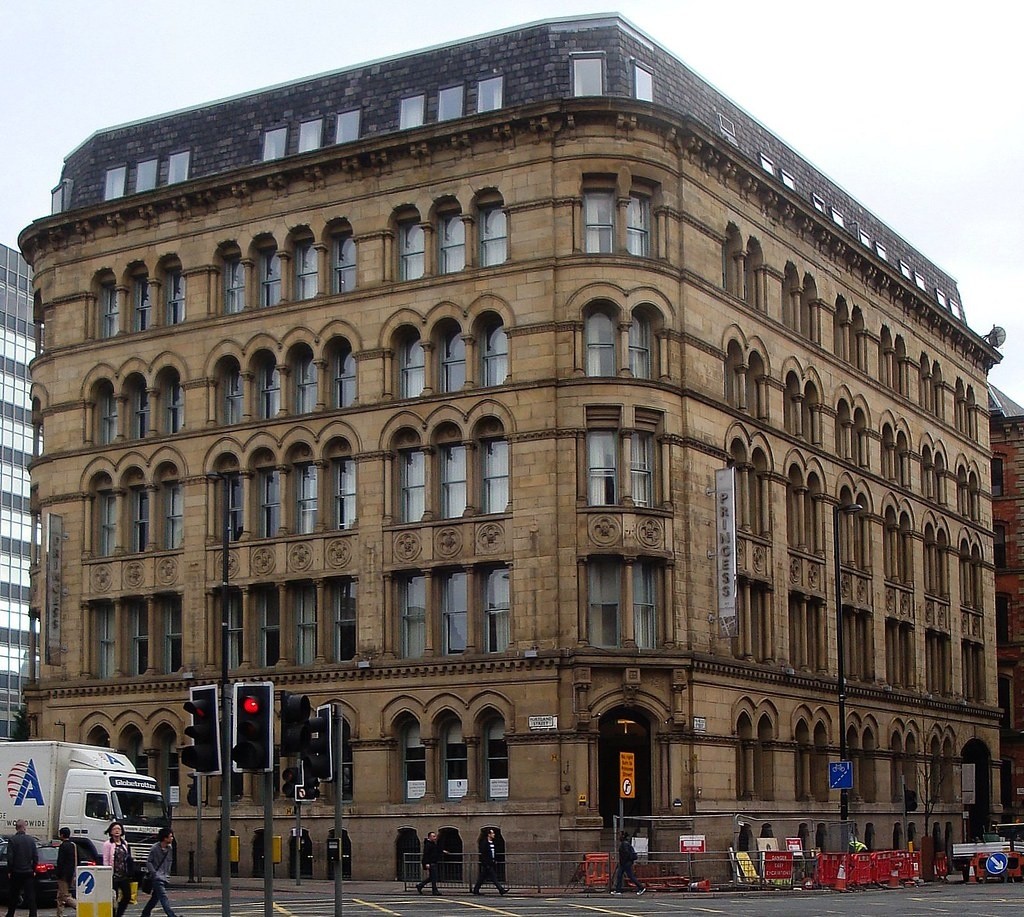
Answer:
[832,503,863,854]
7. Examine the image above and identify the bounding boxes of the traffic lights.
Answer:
[279,689,312,756]
[181,684,223,776]
[300,704,333,782]
[186,772,201,806]
[232,683,274,773]
[282,766,300,799]
[294,760,321,801]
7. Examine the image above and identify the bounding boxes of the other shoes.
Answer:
[474,889,484,896]
[416,885,422,893]
[500,888,509,895]
[432,891,442,896]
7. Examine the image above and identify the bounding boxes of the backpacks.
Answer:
[628,845,637,861]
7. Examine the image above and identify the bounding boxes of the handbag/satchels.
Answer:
[142,873,153,894]
[128,881,138,904]
[71,872,76,887]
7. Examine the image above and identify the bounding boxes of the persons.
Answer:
[5,819,39,917]
[103,822,131,917]
[416,832,448,895]
[140,828,177,917]
[56,828,76,917]
[611,830,646,895]
[473,829,508,896]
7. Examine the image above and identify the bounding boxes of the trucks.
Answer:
[0,739,172,887]
[952,822,1024,883]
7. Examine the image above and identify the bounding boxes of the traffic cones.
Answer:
[882,868,905,889]
[829,856,850,892]
[687,879,711,893]
[966,861,978,885]
[934,864,939,882]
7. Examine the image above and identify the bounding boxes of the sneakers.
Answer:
[611,890,622,895]
[637,887,646,894]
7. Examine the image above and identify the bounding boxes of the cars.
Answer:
[0,839,77,910]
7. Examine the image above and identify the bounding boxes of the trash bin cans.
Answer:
[983,832,999,842]
